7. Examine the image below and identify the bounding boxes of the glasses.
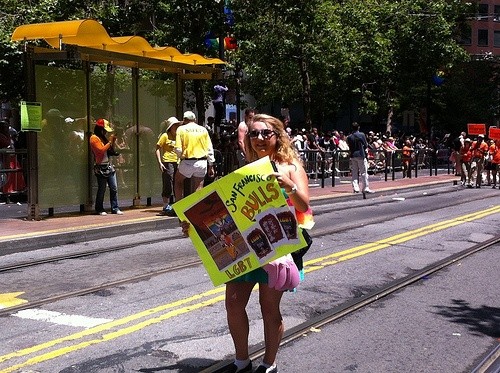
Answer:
[249,130,275,138]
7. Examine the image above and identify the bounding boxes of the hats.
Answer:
[461,132,466,136]
[65,118,74,123]
[478,134,485,138]
[96,119,114,132]
[369,131,373,133]
[46,109,64,118]
[465,138,470,142]
[389,137,393,140]
[183,111,196,119]
[166,117,183,132]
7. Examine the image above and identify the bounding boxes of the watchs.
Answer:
[285,184,297,196]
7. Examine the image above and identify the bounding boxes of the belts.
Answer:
[181,158,207,161]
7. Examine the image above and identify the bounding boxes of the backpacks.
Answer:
[454,138,462,151]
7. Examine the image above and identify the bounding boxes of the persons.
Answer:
[212,79,228,126]
[34,109,167,199]
[89,119,124,215]
[155,116,181,216]
[175,111,215,202]
[0,117,29,204]
[179,114,315,373]
[204,108,500,199]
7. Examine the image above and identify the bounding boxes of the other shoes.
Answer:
[97,211,107,215]
[163,208,177,216]
[212,360,252,373]
[254,361,277,372]
[353,190,359,193]
[461,181,500,189]
[112,209,123,215]
[362,188,373,193]
[334,168,340,173]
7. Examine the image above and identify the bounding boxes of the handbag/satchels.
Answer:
[93,162,115,177]
[292,229,312,261]
